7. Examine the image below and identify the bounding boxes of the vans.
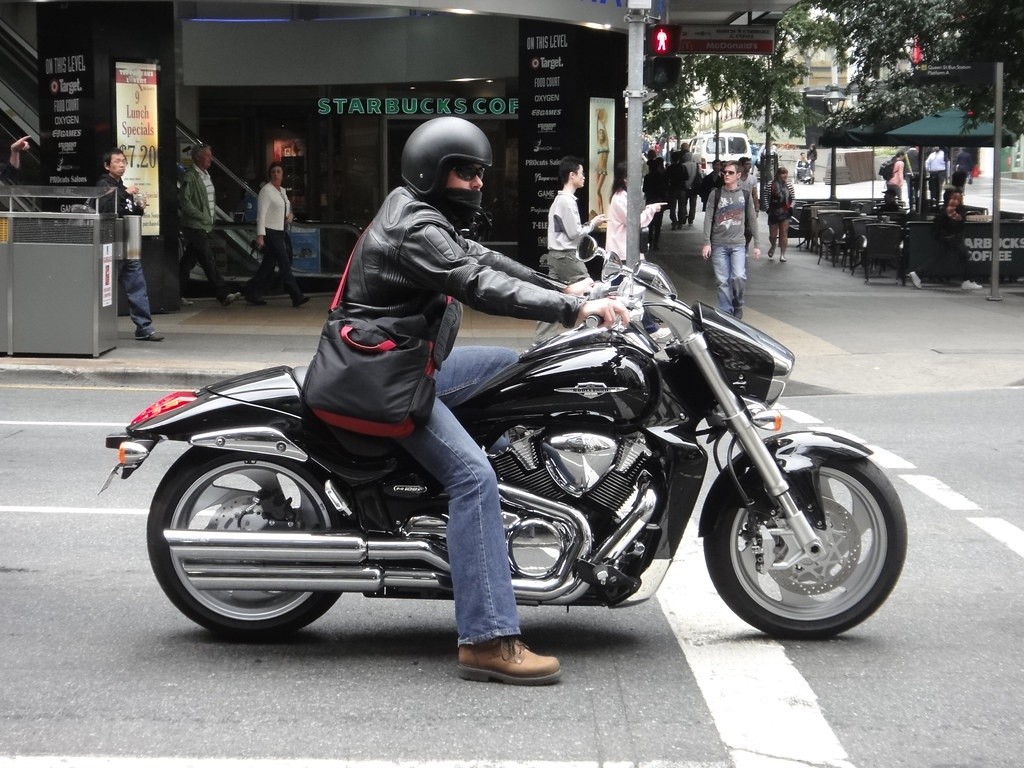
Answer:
[688,132,753,182]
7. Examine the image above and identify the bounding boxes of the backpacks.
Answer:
[879,160,902,180]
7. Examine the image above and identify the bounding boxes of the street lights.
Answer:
[823,83,847,201]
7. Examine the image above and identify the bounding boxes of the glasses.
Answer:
[450,164,486,182]
[723,171,735,175]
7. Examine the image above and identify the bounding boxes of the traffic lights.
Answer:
[644,22,683,89]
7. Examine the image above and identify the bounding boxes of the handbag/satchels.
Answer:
[302,307,439,438]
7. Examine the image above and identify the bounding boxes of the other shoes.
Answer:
[293,296,311,307]
[768,248,775,257]
[731,299,744,320]
[780,256,786,261]
[243,287,267,306]
[147,333,165,341]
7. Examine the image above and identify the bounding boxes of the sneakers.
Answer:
[962,280,983,290]
[909,271,923,289]
[181,298,193,305]
[222,291,241,306]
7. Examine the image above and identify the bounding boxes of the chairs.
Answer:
[791,201,984,286]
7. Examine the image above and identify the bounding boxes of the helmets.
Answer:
[401,116,492,202]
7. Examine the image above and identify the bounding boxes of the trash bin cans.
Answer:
[0,185,142,358]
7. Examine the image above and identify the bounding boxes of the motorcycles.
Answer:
[97,235,909,643]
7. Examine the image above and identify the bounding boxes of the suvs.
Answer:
[661,139,689,160]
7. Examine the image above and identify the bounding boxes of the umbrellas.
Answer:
[816,102,1014,219]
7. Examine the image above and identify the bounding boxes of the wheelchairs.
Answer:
[793,169,814,185]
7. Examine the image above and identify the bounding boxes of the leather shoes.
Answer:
[458,637,563,685]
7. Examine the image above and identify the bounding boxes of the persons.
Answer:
[525,157,607,345]
[299,115,634,687]
[638,134,983,320]
[91,147,165,342]
[604,161,670,345]
[169,142,240,306]
[0,136,31,186]
[242,161,310,310]
